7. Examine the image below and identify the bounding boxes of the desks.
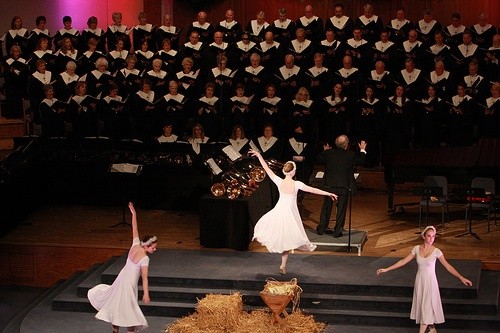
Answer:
[199,171,272,252]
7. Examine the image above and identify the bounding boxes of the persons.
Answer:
[315,135,367,237]
[0,4,500,209]
[88,202,158,333]
[248,149,339,274]
[376,225,472,333]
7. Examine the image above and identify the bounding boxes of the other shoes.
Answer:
[334,233,343,238]
[317,225,322,235]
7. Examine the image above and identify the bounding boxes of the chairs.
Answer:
[464,177,498,232]
[419,176,448,227]
